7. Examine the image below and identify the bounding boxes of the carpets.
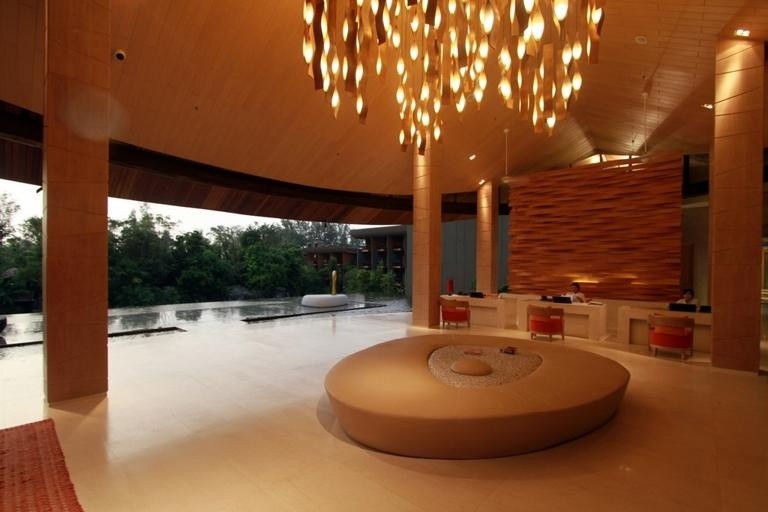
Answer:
[1,419,86,512]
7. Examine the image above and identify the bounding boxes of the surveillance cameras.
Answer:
[113,49,126,62]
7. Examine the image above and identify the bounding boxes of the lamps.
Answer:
[289,0,606,158]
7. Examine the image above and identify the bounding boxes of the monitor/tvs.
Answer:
[553,296,572,304]
[470,291,484,298]
[668,303,696,312]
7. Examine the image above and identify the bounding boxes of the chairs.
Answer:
[439,295,470,328]
[527,304,564,340]
[645,311,696,363]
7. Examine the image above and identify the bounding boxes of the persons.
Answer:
[564,282,586,303]
[676,287,701,310]
